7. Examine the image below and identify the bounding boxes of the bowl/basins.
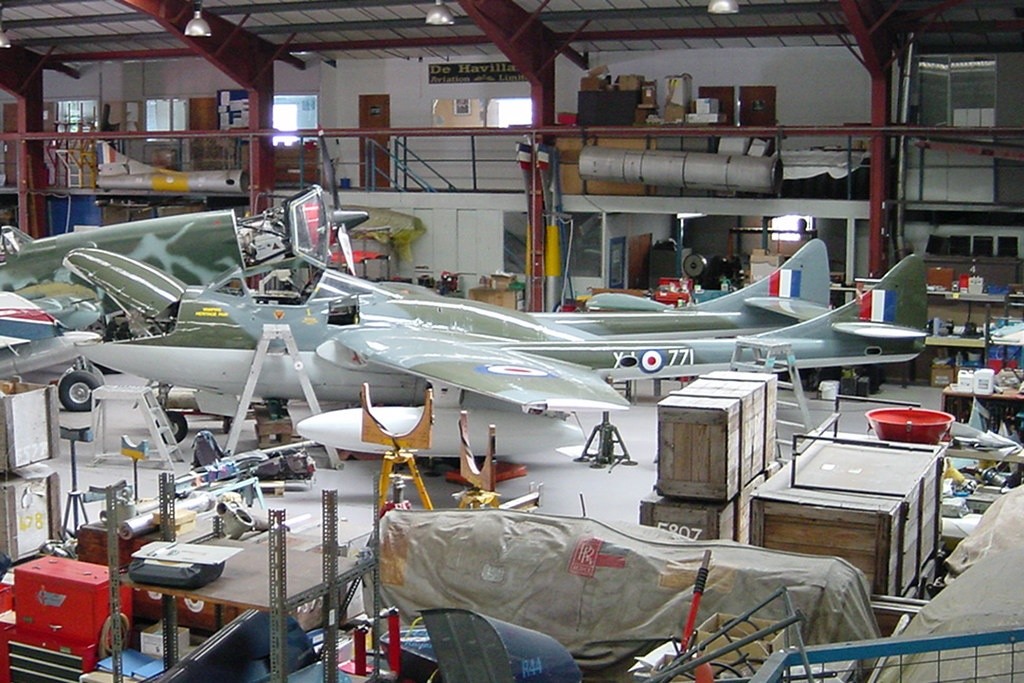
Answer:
[865,405,955,445]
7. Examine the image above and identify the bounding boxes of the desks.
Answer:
[468,286,526,313]
[941,383,1024,432]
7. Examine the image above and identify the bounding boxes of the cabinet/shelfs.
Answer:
[940,440,1024,512]
[829,285,1024,387]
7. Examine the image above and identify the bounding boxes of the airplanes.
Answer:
[0,132,930,471]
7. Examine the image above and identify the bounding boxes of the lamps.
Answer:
[707,0,740,15]
[425,0,455,26]
[0,0,12,49]
[184,0,211,37]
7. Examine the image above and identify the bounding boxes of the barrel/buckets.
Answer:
[819,380,840,401]
[974,368,995,395]
[957,369,974,393]
[988,358,1018,374]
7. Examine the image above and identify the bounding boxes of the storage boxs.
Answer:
[0,380,64,562]
[13,557,134,642]
[490,274,517,290]
[140,623,191,658]
[688,612,786,670]
[931,367,954,387]
[639,368,948,601]
[927,267,955,291]
[575,66,727,126]
